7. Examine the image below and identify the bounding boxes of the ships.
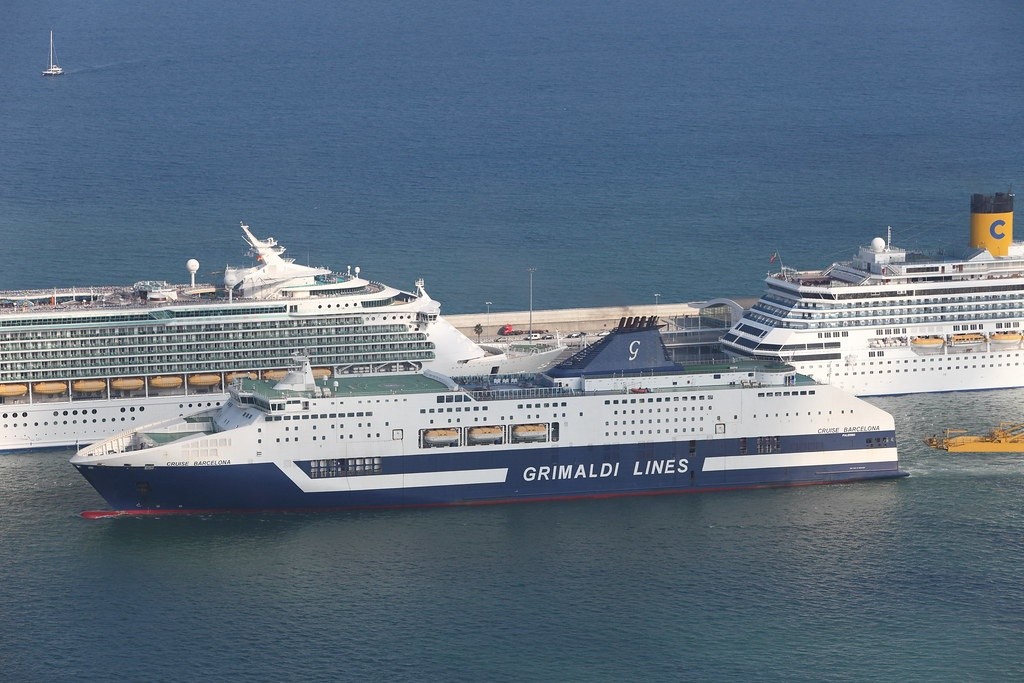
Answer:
[1,223,493,459]
[707,191,1024,398]
[69,313,907,517]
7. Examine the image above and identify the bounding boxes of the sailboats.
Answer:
[40,31,63,75]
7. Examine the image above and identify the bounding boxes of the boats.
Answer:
[988,332,1023,344]
[1,383,26,397]
[425,427,460,448]
[910,336,944,348]
[512,423,545,442]
[264,369,291,380]
[151,374,181,387]
[949,333,986,346]
[33,382,68,395]
[188,374,221,386]
[72,379,106,392]
[309,368,332,379]
[110,377,144,390]
[225,370,256,381]
[468,426,502,444]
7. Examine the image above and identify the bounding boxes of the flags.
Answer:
[770,250,777,263]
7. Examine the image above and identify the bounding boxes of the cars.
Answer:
[482,323,614,345]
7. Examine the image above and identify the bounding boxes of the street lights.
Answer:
[654,292,661,316]
[526,268,537,345]
[486,301,493,340]
